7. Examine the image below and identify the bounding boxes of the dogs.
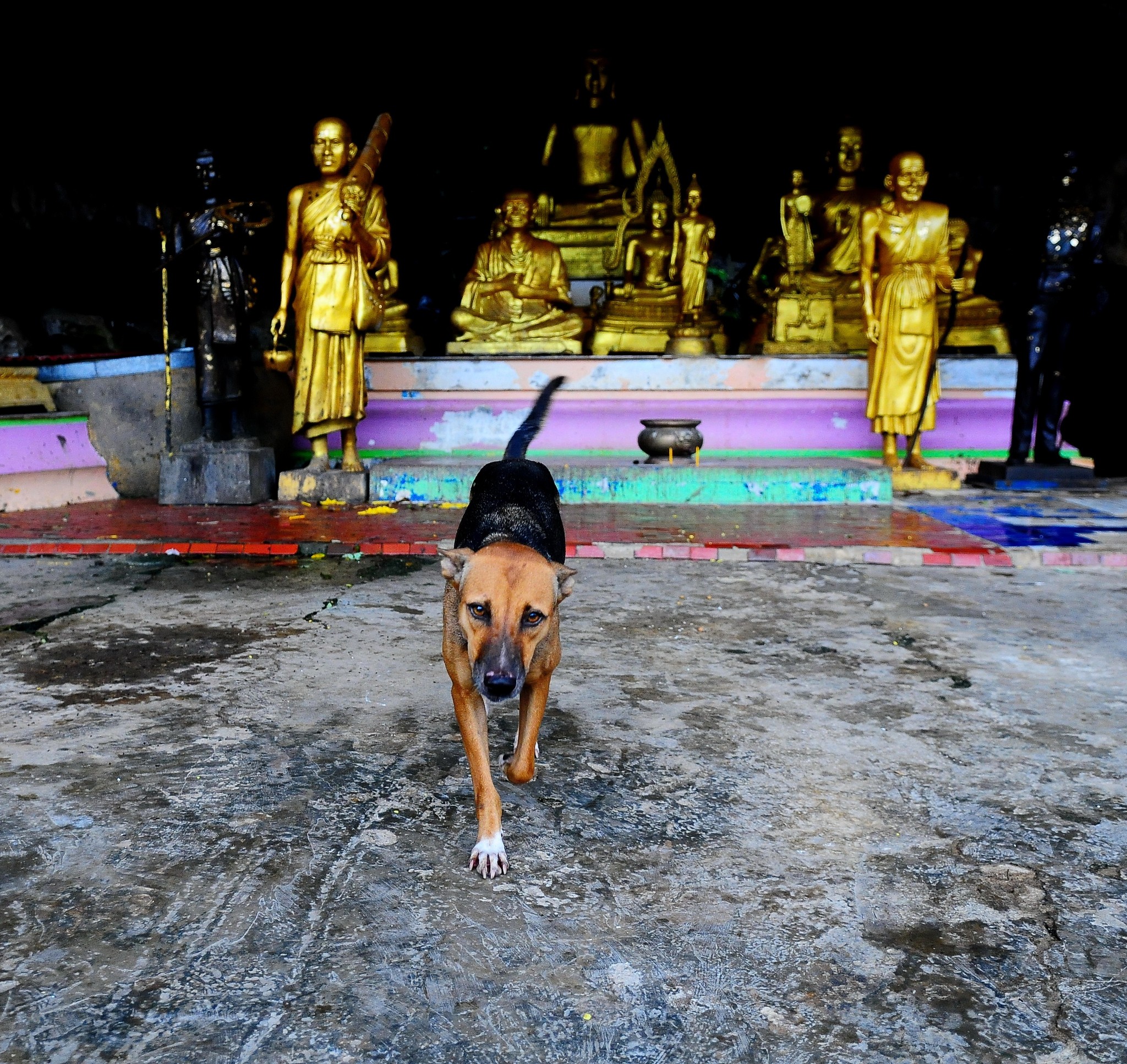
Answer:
[431,373,584,883]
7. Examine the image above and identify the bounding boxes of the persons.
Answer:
[780,169,816,293]
[609,194,686,299]
[528,48,663,231]
[450,191,585,340]
[269,118,393,473]
[155,147,276,443]
[936,215,982,299]
[668,173,717,320]
[812,127,882,273]
[1006,147,1111,468]
[858,151,967,474]
